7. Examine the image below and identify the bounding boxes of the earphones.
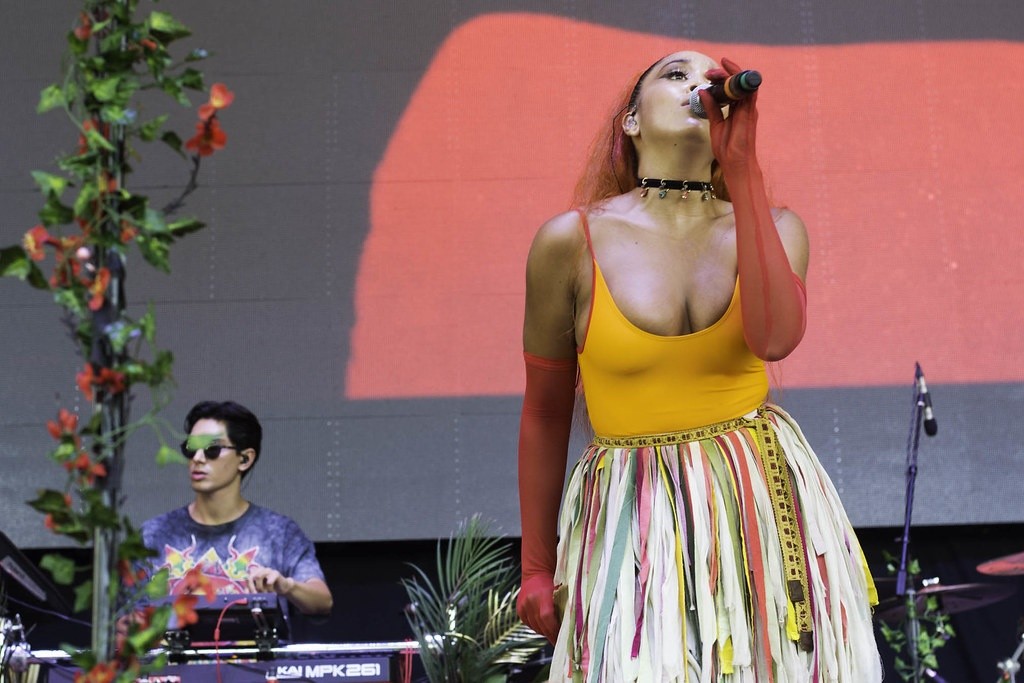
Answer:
[242,455,249,464]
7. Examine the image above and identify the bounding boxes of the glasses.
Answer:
[180,440,239,460]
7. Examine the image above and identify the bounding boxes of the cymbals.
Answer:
[875,580,1013,620]
[975,552,1024,578]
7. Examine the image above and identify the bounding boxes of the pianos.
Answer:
[37,643,411,683]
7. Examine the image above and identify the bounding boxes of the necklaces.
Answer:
[634,177,717,201]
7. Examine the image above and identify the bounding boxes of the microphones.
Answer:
[690,70,763,119]
[916,366,937,436]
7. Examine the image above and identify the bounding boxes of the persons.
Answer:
[513,51,884,683]
[115,400,333,645]
